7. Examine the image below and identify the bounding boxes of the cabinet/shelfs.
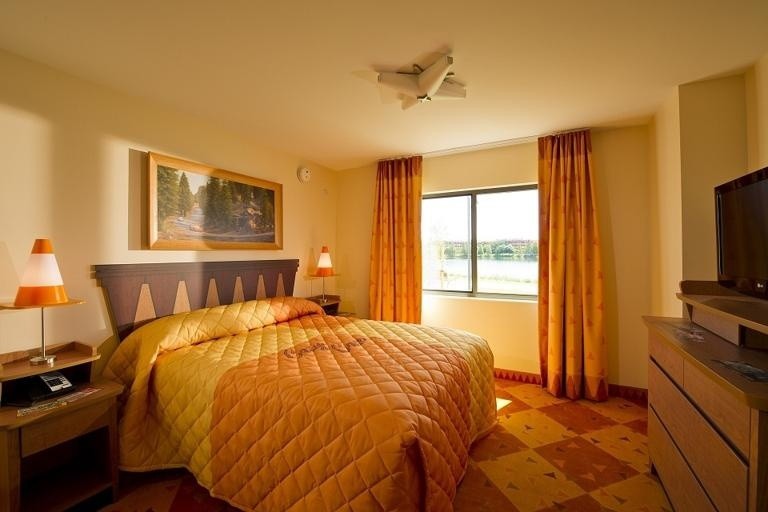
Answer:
[642,296,768,512]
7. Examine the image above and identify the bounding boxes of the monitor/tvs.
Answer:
[713,165,767,301]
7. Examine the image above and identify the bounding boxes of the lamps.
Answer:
[309,245,341,304]
[377,55,467,111]
[0,239,85,367]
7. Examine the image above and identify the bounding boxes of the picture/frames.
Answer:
[146,150,284,251]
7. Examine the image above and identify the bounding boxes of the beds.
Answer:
[91,259,497,512]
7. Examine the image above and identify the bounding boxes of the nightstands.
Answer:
[0,346,125,512]
[313,299,340,315]
